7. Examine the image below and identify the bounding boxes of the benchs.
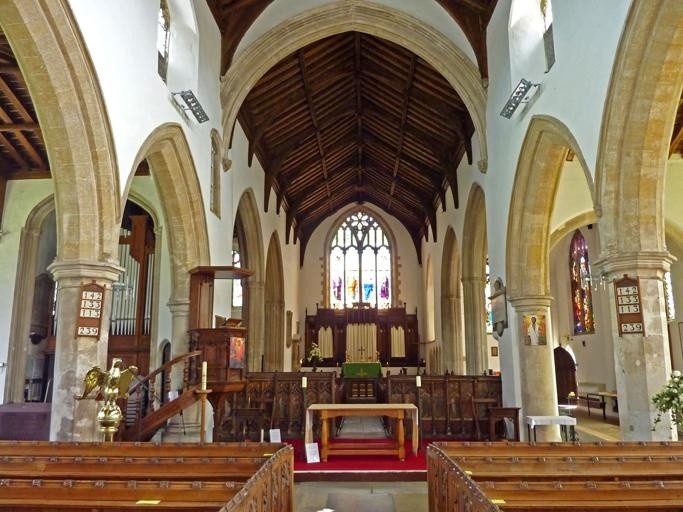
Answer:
[0,434,301,512]
[420,433,682,510]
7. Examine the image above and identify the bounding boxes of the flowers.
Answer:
[642,366,683,434]
[305,340,323,364]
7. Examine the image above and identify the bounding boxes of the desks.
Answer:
[486,405,522,441]
[308,403,419,465]
[229,408,264,440]
[586,390,616,421]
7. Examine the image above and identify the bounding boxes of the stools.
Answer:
[526,415,578,441]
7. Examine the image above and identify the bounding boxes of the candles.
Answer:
[300,376,307,388]
[415,375,422,387]
[199,360,207,390]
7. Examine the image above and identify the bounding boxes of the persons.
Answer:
[527,316,541,345]
